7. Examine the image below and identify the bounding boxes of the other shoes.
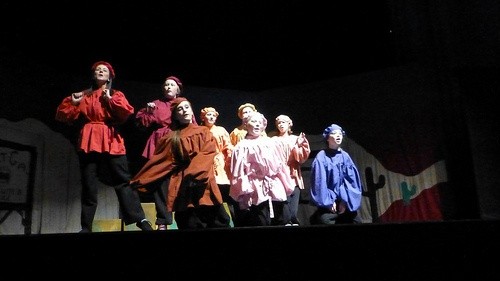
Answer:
[158,225,167,230]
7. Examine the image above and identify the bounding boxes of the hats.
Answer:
[92,61,114,78]
[238,103,255,119]
[323,124,345,139]
[276,115,292,127]
[166,76,182,91]
[200,107,219,120]
[243,111,267,129]
[170,97,187,112]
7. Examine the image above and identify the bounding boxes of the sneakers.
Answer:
[78,229,92,233]
[136,218,153,231]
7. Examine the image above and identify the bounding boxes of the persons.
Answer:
[271,114,311,226]
[127,98,230,230]
[199,106,234,230]
[55,60,156,233]
[135,75,197,230]
[229,103,268,147]
[309,124,362,225]
[229,113,295,227]
